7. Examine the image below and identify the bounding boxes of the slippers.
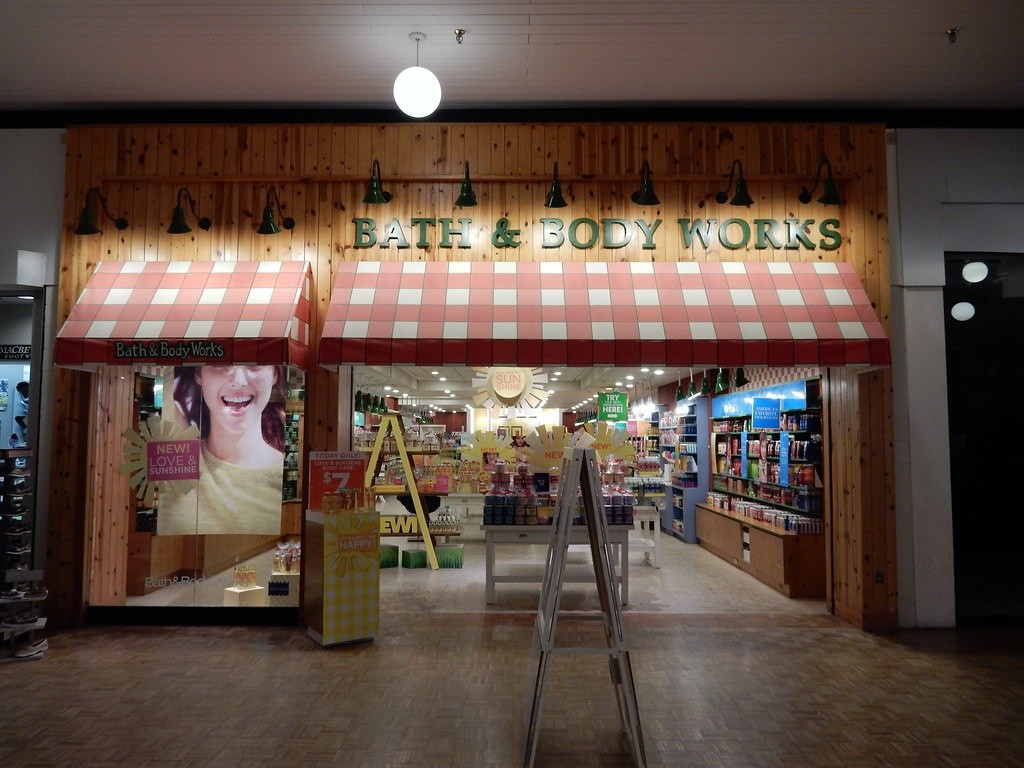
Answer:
[16,562,28,571]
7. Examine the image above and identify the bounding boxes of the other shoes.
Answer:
[16,638,49,657]
[3,614,39,626]
[9,433,19,445]
[7,467,30,477]
[3,505,29,516]
[14,542,30,551]
[1,582,48,597]
[6,481,32,496]
[8,522,31,533]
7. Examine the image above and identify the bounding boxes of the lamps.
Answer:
[574,405,597,426]
[371,385,379,415]
[713,367,729,394]
[257,188,295,234]
[74,188,127,234]
[716,160,754,206]
[287,367,292,400]
[167,187,211,233]
[361,159,392,205]
[455,162,480,207]
[734,368,749,389]
[544,161,569,209]
[379,386,389,415]
[631,160,661,206]
[365,383,373,413]
[700,368,713,396]
[298,370,306,401]
[355,387,364,413]
[799,158,842,206]
[391,31,442,119]
[421,406,434,424]
[674,370,686,401]
[686,368,700,401]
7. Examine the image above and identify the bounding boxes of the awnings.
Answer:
[53,260,312,374]
[317,260,892,369]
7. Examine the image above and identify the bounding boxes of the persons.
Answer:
[483,452,499,465]
[158,363,284,535]
[509,436,530,447]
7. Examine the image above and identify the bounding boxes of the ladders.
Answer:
[521,447,649,767]
[364,413,439,571]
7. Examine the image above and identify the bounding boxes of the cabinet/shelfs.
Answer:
[694,502,824,598]
[360,432,463,537]
[707,375,822,536]
[661,392,709,543]
[0,448,49,664]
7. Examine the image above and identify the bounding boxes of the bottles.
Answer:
[460,471,472,482]
[273,552,300,574]
[422,464,452,485]
[234,564,255,589]
[322,487,376,515]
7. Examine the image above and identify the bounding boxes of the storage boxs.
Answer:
[268,571,299,607]
[435,543,464,569]
[379,543,399,569]
[224,586,264,607]
[402,549,427,569]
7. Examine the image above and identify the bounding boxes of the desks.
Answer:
[480,523,634,604]
